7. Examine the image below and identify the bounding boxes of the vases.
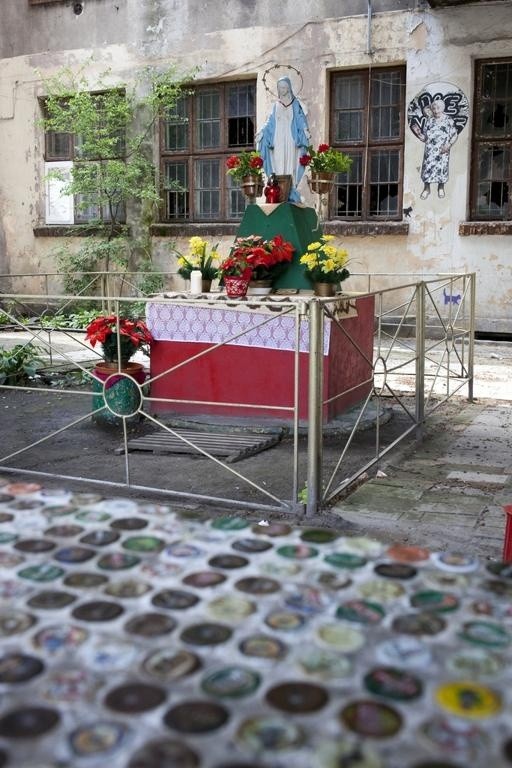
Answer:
[310,170,336,195]
[241,172,265,197]
[105,351,131,369]
[185,269,338,297]
[265,179,281,203]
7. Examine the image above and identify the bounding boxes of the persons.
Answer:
[254,76,312,203]
[411,100,458,200]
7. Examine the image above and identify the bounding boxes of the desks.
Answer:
[145,290,375,425]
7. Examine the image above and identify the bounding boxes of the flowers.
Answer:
[84,313,155,361]
[171,233,363,283]
[225,149,267,181]
[298,142,353,175]
